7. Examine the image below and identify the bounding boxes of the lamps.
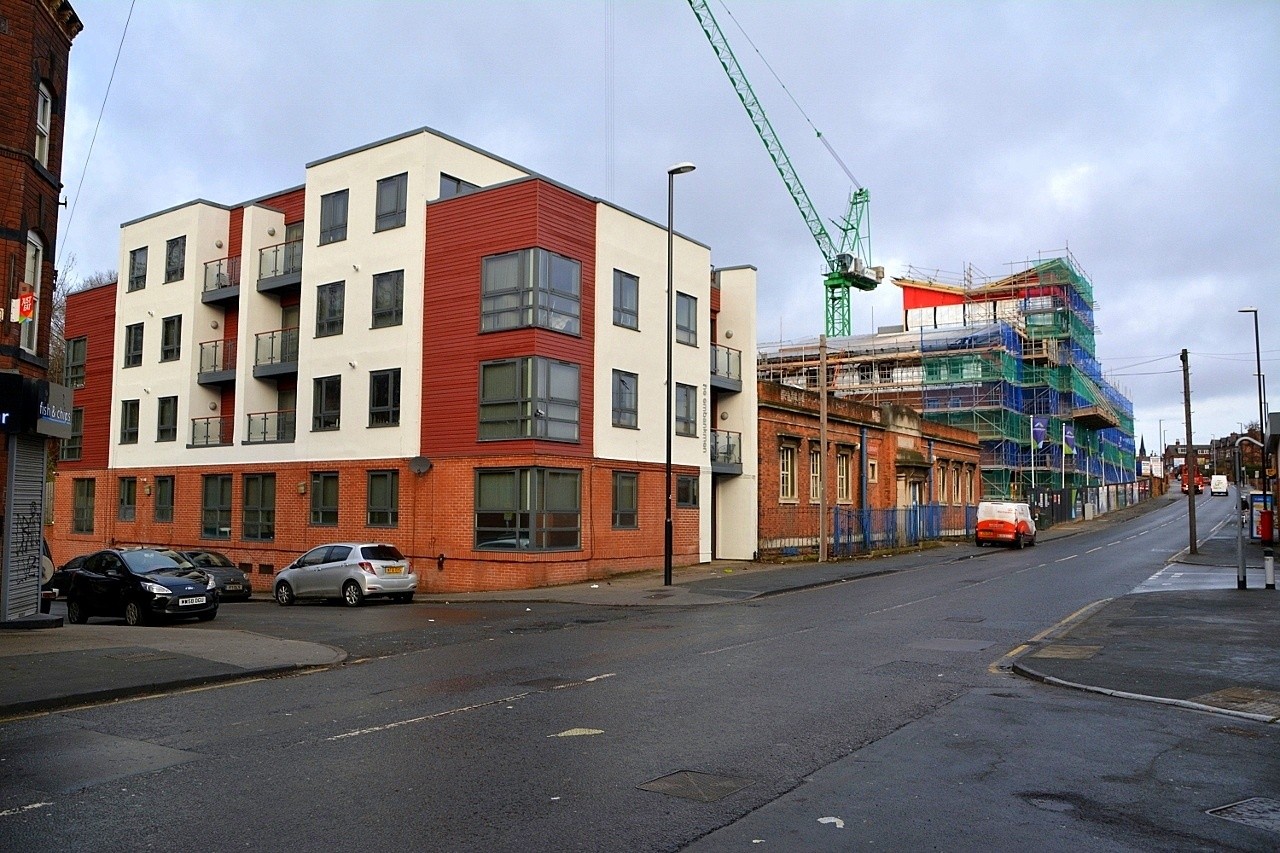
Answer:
[143,387,150,395]
[143,484,151,496]
[726,330,733,338]
[147,310,155,317]
[211,321,219,329]
[297,482,306,493]
[215,240,223,248]
[721,411,728,420]
[352,264,359,271]
[208,401,217,410]
[267,227,276,236]
[349,362,357,368]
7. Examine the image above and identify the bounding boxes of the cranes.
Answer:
[691,0,884,343]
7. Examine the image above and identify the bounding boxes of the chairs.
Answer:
[718,443,736,464]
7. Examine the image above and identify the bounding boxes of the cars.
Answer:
[1177,474,1182,480]
[50,552,95,603]
[1202,477,1210,485]
[272,540,417,607]
[64,546,220,627]
[176,549,253,602]
[480,537,529,550]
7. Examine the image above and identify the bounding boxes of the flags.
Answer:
[1065,425,1078,454]
[1033,416,1049,449]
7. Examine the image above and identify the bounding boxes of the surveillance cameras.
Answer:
[536,410,544,415]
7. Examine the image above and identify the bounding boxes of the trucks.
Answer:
[1211,474,1230,495]
[1180,463,1204,495]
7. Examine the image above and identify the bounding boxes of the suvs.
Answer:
[41,536,60,615]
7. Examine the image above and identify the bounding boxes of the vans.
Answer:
[974,500,1039,549]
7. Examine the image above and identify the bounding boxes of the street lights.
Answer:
[660,162,697,586]
[1237,309,1269,509]
[1158,419,1165,462]
[1237,422,1244,467]
[1163,429,1168,459]
[1253,372,1267,428]
[1210,433,1217,475]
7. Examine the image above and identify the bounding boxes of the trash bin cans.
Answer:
[1085,503,1095,521]
[1034,513,1046,531]
